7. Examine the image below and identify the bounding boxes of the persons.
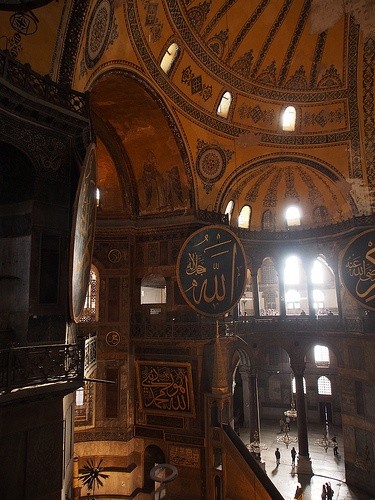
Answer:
[294,486,304,500]
[260,309,280,316]
[275,448,281,467]
[301,310,306,315]
[331,436,337,442]
[323,428,328,441]
[328,311,333,315]
[240,311,248,323]
[321,482,332,500]
[291,447,297,465]
[333,446,338,455]
[279,416,291,440]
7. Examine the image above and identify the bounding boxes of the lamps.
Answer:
[283,393,298,417]
[78,457,109,491]
